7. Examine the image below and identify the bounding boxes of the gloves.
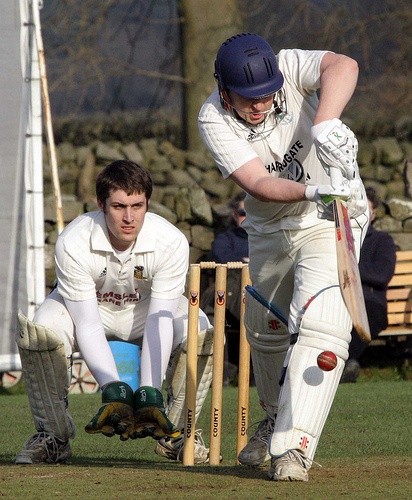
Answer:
[85,381,134,441]
[311,120,358,180]
[121,387,174,441]
[308,185,367,221]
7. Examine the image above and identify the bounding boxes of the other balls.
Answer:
[317,351,337,371]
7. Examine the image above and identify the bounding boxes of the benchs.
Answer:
[359,248,412,342]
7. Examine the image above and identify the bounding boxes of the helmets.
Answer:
[216,32,284,100]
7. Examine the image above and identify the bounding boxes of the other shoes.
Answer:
[340,361,360,382]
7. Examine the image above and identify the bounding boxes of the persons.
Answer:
[16,161,224,466]
[195,186,264,373]
[338,183,396,383]
[196,33,368,482]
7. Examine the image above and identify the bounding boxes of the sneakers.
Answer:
[268,450,323,482]
[14,434,72,465]
[239,416,276,465]
[154,429,223,464]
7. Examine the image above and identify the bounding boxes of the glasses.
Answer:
[235,208,246,216]
